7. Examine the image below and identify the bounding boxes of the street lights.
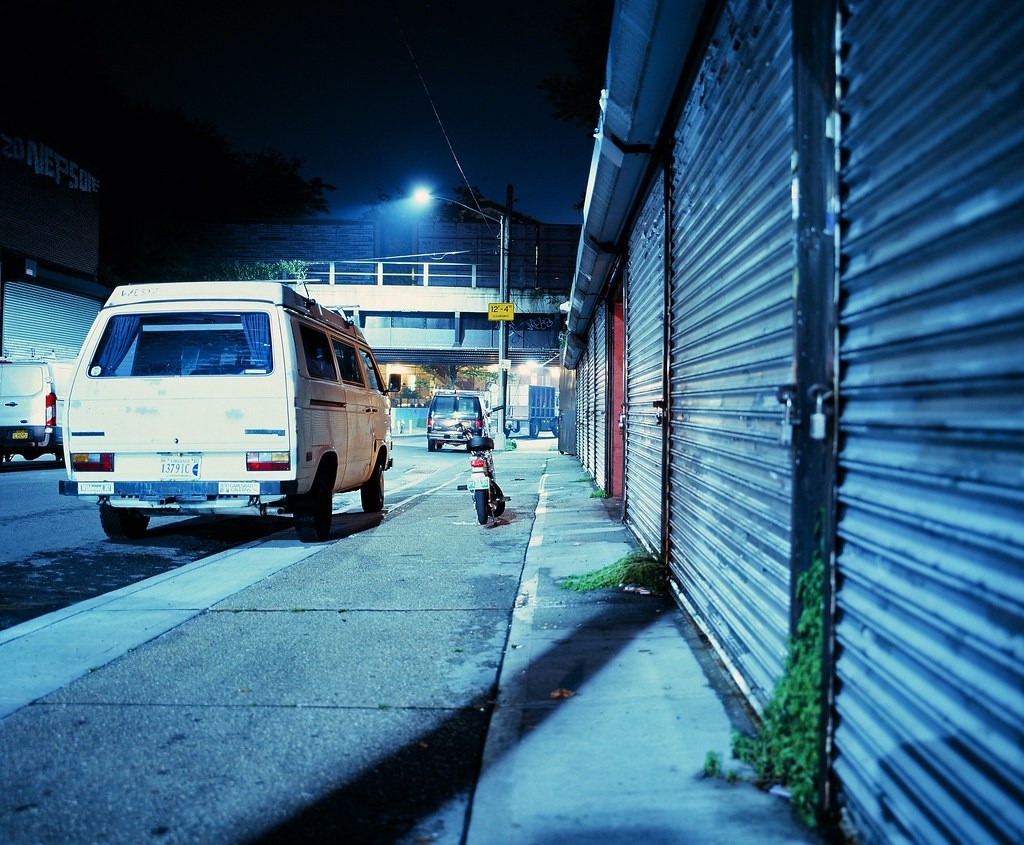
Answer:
[412,190,507,449]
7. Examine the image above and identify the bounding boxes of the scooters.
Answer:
[460,403,509,528]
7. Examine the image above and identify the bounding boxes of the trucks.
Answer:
[491,385,562,439]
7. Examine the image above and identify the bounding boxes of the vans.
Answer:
[427,389,489,452]
[58,280,402,543]
[0,351,79,467]
